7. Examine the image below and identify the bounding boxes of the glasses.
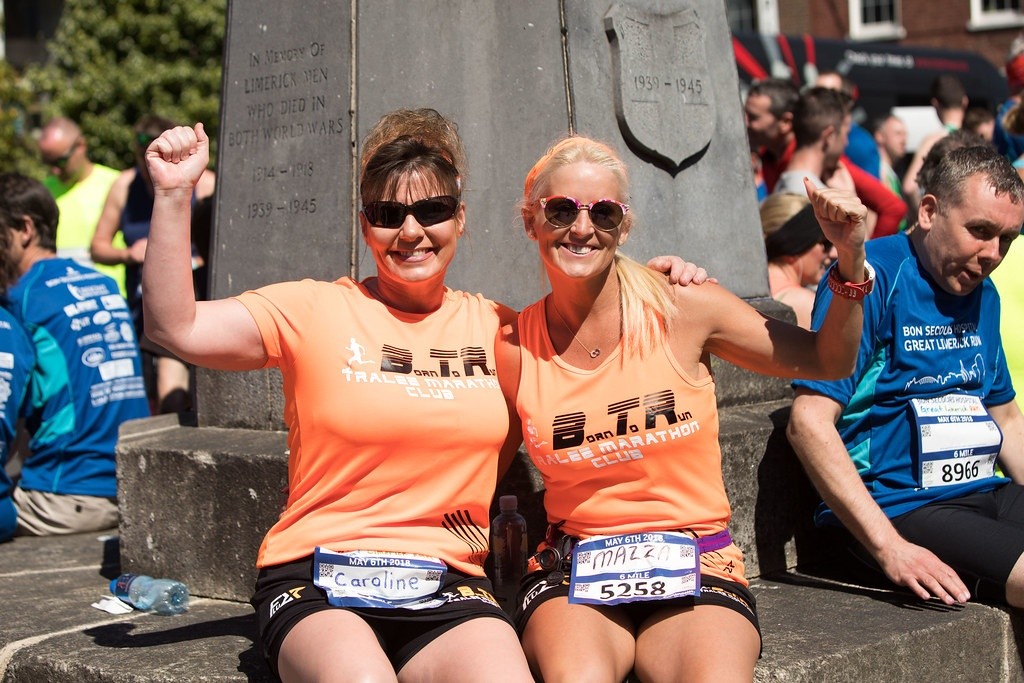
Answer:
[535,195,630,232]
[364,195,460,228]
[41,139,79,167]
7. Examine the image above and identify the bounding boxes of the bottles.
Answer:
[490,496,528,623]
[109,573,190,616]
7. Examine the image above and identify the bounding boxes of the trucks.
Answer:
[731,34,1010,129]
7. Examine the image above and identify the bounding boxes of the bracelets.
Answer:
[192,258,199,271]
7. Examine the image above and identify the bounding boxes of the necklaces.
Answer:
[549,298,622,359]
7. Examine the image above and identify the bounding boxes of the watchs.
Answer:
[829,259,875,301]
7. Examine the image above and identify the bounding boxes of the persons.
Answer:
[743,45,1024,478]
[784,146,1024,607]
[0,118,217,539]
[494,138,868,683]
[142,107,721,683]
